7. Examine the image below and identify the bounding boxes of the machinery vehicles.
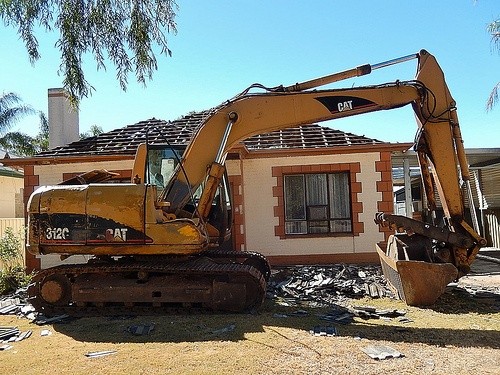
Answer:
[26,47,487,318]
